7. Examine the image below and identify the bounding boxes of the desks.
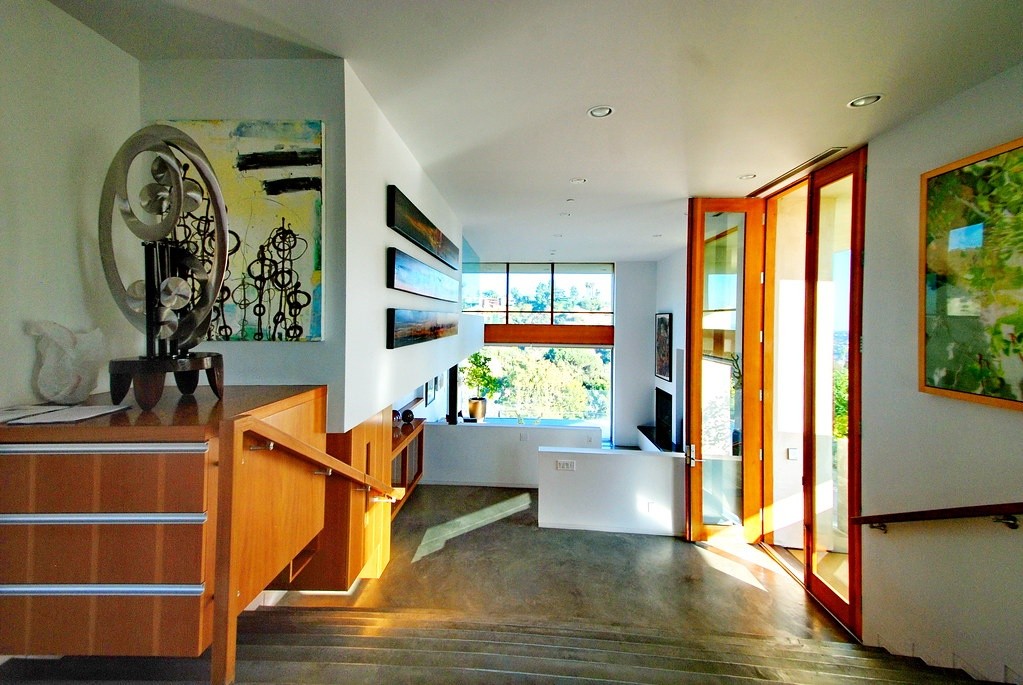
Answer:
[0,383,328,658]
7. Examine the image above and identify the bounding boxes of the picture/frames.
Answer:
[655,313,673,382]
[917,136,1023,410]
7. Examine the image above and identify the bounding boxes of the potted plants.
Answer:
[459,353,505,422]
[833,413,848,533]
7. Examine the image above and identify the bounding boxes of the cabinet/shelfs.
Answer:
[390,418,427,521]
